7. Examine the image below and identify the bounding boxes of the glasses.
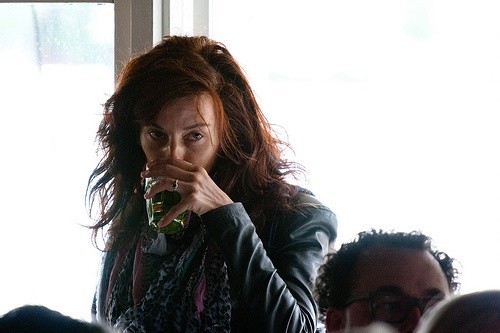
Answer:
[331,290,428,323]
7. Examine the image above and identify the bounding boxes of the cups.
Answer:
[145,159,189,233]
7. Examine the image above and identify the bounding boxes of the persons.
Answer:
[415,290,500,333]
[310,228,461,333]
[0,305,115,333]
[81,36,339,333]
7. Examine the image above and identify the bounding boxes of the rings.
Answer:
[172,179,179,192]
